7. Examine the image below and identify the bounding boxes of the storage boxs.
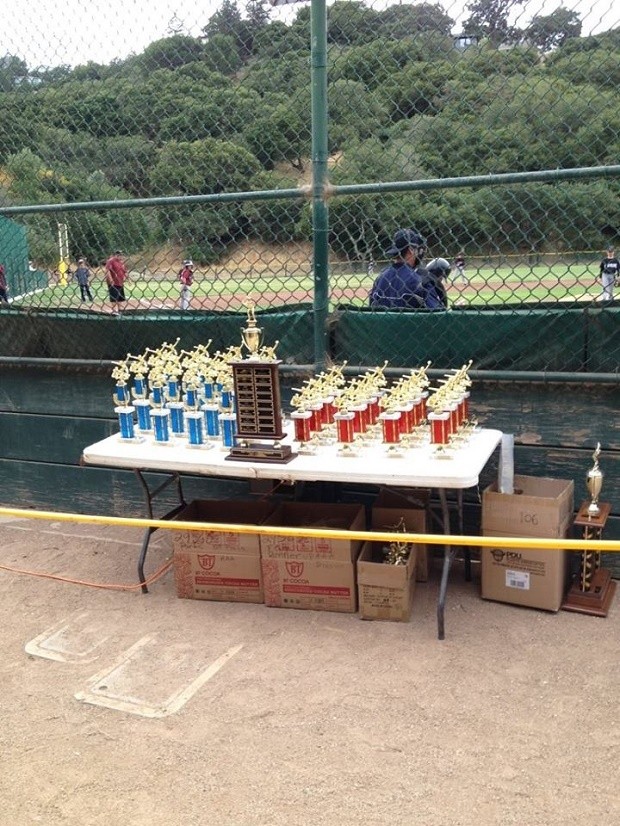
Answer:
[171,481,432,623]
[481,474,575,611]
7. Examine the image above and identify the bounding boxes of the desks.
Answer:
[83,412,505,640]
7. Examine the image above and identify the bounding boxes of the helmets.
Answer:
[426,257,451,283]
[385,229,419,254]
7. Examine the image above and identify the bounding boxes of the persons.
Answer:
[0,263,10,304]
[74,258,94,303]
[598,245,620,301]
[104,250,132,315]
[424,258,451,307]
[177,260,195,310]
[368,228,447,312]
[28,260,38,272]
[451,252,467,286]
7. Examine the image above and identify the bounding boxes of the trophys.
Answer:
[224,291,298,465]
[288,359,482,461]
[109,336,291,453]
[559,440,618,619]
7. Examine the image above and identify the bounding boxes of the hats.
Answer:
[608,246,614,252]
[184,260,193,266]
